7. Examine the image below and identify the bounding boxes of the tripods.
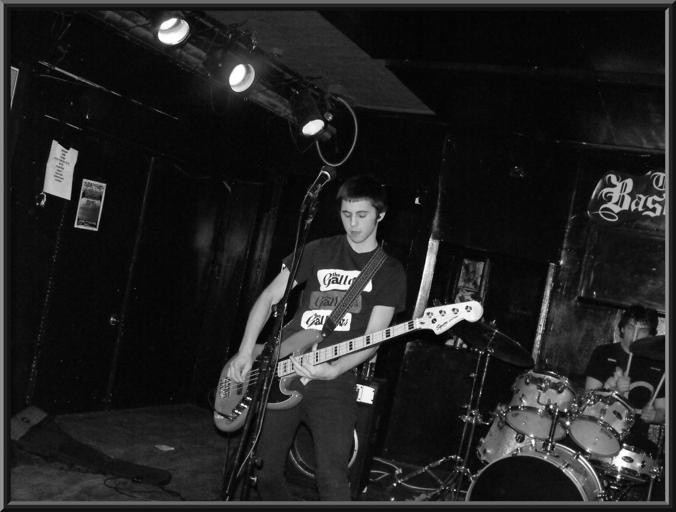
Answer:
[385,348,489,501]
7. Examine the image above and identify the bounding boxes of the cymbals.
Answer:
[629,336,665,361]
[452,320,535,368]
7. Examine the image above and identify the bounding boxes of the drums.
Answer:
[584,442,657,483]
[504,369,576,439]
[464,441,604,502]
[477,406,545,464]
[569,389,635,456]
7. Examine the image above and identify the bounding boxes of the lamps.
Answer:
[150,11,336,139]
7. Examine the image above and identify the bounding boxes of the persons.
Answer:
[226,176,407,501]
[584,304,665,437]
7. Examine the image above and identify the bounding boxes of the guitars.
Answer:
[213,296,484,432]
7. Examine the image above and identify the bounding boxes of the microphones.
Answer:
[300,165,337,213]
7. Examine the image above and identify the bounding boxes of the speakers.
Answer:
[285,377,381,501]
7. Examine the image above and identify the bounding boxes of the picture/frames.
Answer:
[455,256,487,292]
